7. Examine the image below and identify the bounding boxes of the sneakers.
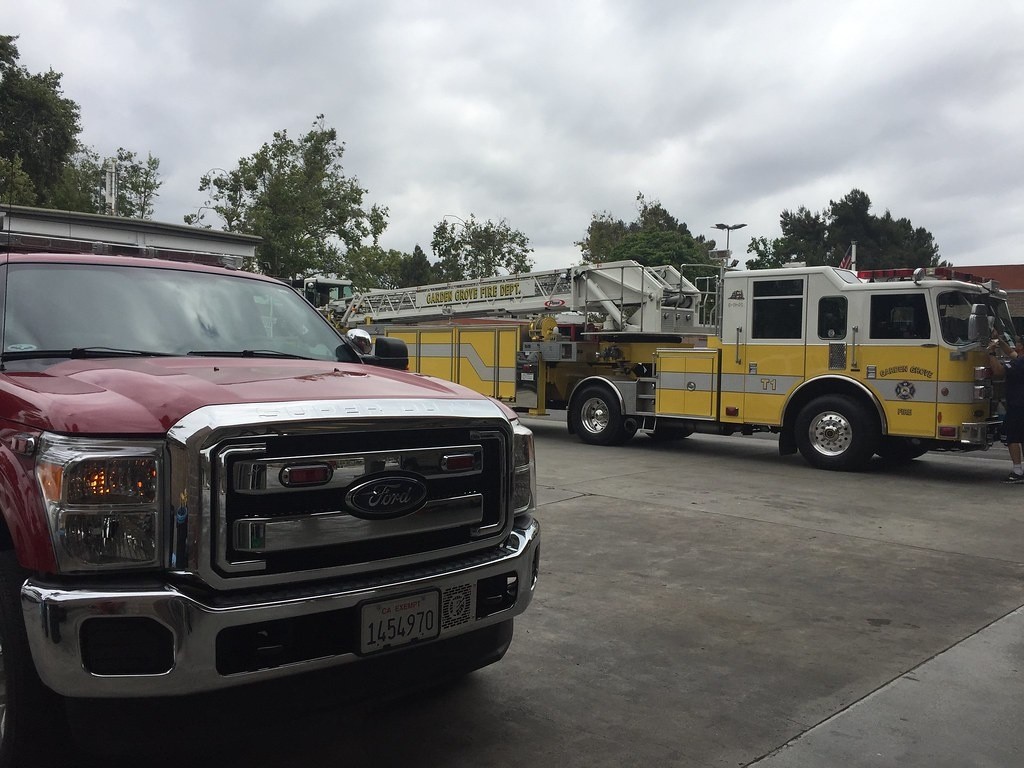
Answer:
[1000,472,1024,484]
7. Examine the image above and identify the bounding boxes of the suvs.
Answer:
[0,204,541,767]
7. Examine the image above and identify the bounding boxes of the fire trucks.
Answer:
[260,260,1018,472]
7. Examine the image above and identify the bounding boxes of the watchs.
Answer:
[989,352,996,356]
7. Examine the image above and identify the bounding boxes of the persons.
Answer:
[987,329,1024,483]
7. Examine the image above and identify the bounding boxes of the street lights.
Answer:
[710,224,747,267]
